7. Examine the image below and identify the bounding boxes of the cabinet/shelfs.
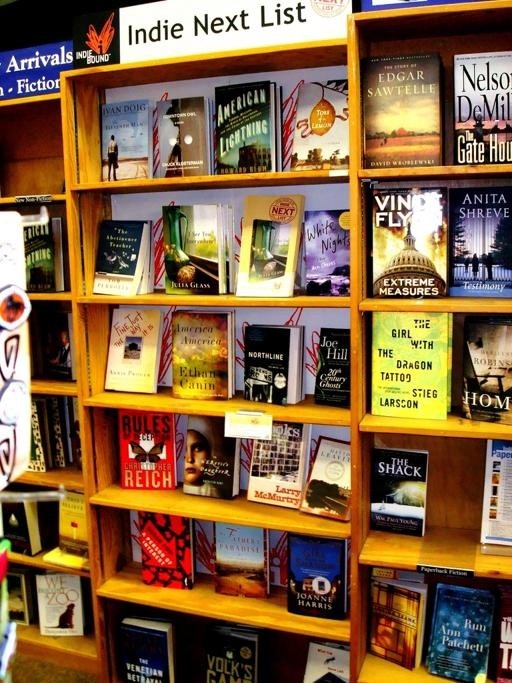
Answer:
[344,0,512,682]
[55,33,357,682]
[0,84,112,683]
